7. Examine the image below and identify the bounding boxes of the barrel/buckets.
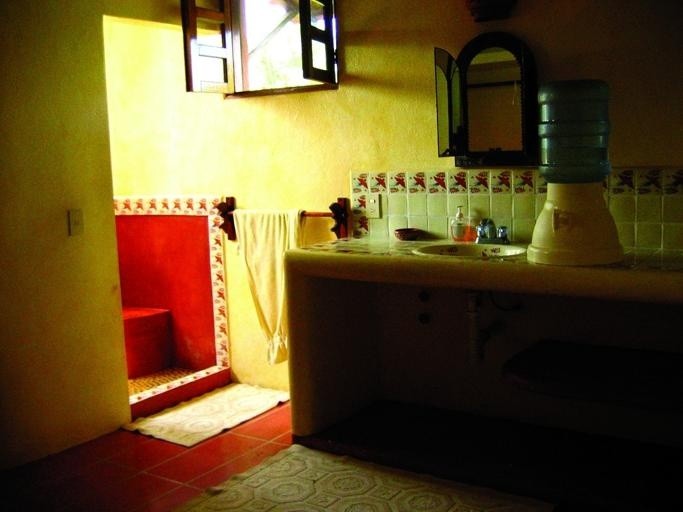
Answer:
[537,79,612,183]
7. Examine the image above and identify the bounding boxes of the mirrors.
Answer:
[435,29,538,168]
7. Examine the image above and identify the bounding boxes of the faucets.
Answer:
[479,219,497,240]
[554,209,568,232]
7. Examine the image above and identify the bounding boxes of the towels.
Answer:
[232,207,306,365]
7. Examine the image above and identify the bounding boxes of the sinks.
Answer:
[417,244,525,258]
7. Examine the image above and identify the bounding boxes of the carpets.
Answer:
[177,445,554,512]
[121,382,291,449]
